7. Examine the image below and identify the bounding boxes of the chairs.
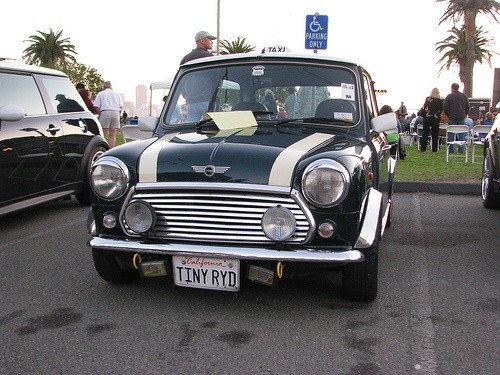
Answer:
[315,99,356,121]
[446,125,469,163]
[230,101,270,120]
[472,125,492,163]
[399,123,479,150]
[121,125,153,144]
[384,127,400,161]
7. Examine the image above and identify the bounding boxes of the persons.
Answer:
[379,83,500,161]
[76,31,331,153]
[55,94,85,113]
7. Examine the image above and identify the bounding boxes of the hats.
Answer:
[195,30,216,42]
[103,80,111,87]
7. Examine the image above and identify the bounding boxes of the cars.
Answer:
[1,63,111,215]
[87,46,399,301]
[480,114,500,210]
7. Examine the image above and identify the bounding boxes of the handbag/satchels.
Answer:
[417,108,427,118]
